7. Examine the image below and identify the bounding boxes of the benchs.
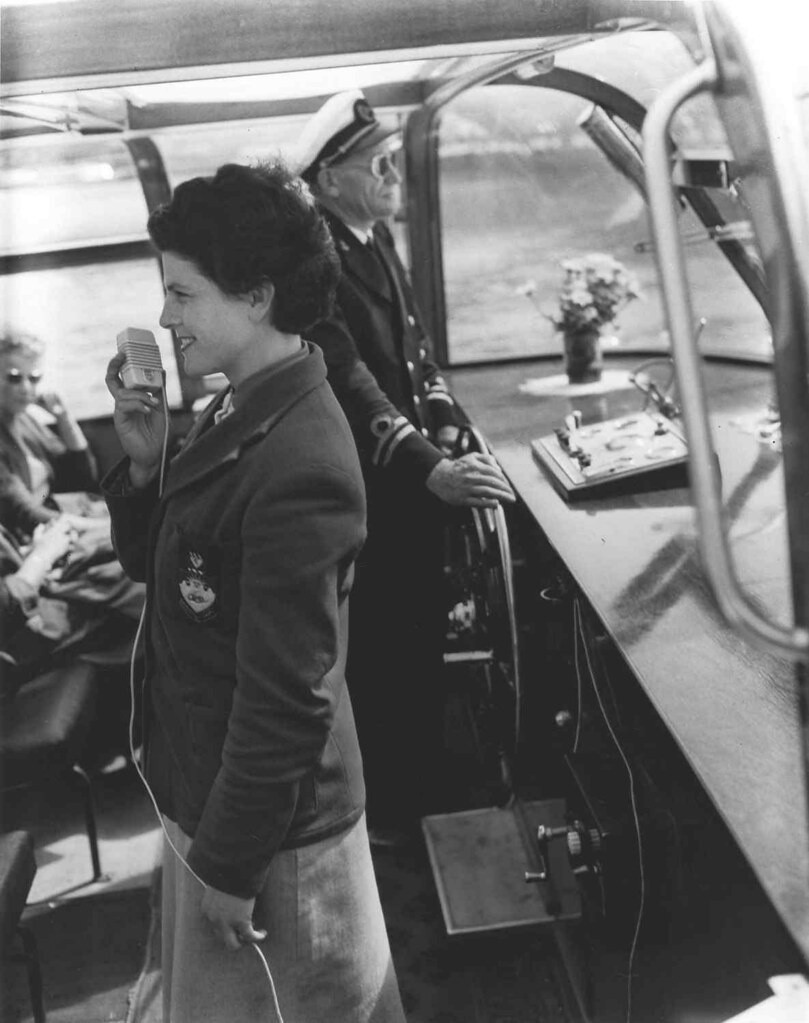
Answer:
[2,660,106,1023]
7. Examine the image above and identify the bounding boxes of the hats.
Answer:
[290,90,402,184]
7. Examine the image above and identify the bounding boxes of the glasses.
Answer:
[326,152,395,178]
[0,369,41,382]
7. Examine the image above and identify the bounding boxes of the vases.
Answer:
[566,325,603,384]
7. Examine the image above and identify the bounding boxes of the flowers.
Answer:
[520,253,639,335]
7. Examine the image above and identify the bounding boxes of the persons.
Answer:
[279,87,515,842]
[1,322,146,690]
[100,163,407,1022]
